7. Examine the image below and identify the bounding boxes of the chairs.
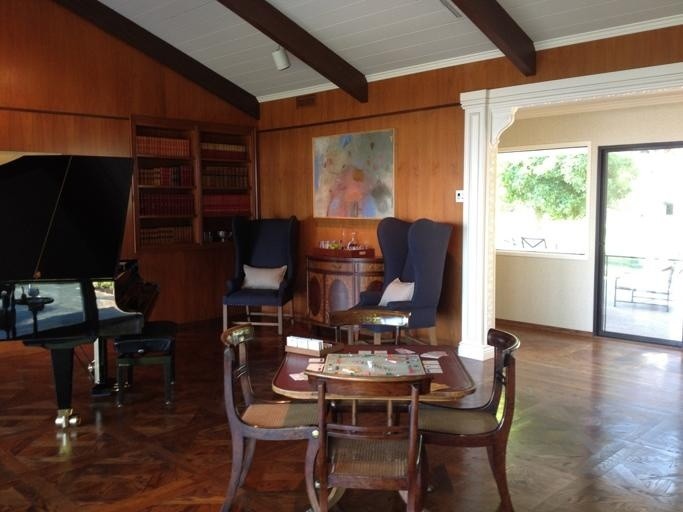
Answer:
[349,217,453,347]
[612,264,675,311]
[221,322,333,512]
[326,309,414,441]
[312,381,428,512]
[408,328,521,512]
[223,213,298,334]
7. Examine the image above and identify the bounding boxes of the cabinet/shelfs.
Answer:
[129,114,198,255]
[197,122,260,248]
[306,257,404,343]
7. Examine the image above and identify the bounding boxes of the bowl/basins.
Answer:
[217,230,232,238]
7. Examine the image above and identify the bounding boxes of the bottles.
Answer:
[348,232,359,250]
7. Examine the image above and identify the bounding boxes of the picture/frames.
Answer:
[311,127,395,220]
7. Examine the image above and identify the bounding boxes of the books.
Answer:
[136,134,252,247]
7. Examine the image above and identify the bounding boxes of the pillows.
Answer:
[379,278,415,308]
[241,265,288,290]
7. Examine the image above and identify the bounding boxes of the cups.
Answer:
[203,231,213,241]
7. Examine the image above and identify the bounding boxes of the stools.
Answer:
[114,320,178,408]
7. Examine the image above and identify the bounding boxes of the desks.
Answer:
[272,345,476,512]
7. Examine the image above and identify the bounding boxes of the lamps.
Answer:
[271,45,292,71]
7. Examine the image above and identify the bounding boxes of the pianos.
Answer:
[0,155,160,428]
